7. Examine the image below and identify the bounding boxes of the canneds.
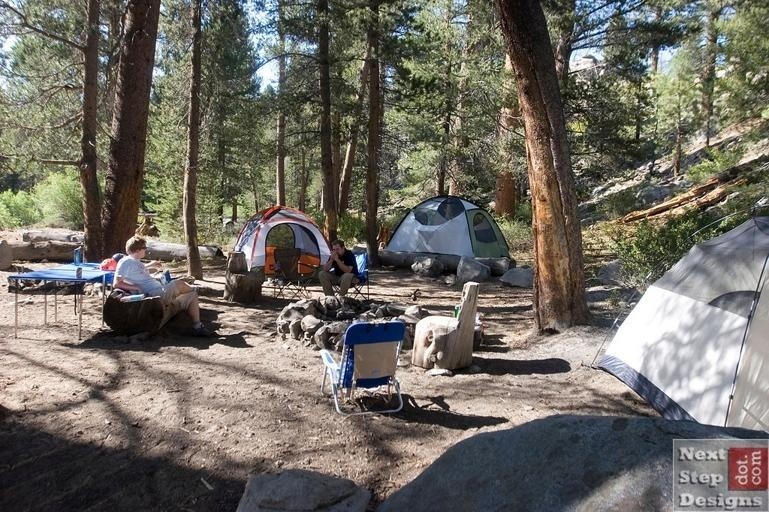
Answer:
[73,249,80,265]
[75,267,83,278]
[455,305,461,318]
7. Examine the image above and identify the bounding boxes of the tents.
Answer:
[581,204,769,433]
[383,194,511,258]
[233,205,335,275]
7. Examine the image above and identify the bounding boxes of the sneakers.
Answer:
[191,323,217,338]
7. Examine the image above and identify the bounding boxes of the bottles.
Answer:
[454,302,462,319]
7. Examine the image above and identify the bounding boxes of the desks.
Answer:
[8,262,115,342]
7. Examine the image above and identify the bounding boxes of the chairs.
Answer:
[270,247,319,300]
[318,319,406,416]
[410,281,479,370]
[334,248,369,304]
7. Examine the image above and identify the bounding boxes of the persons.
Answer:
[111,235,217,337]
[318,239,360,304]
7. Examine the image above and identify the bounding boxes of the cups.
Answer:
[74,248,81,266]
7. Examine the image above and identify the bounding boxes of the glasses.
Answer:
[140,246,148,250]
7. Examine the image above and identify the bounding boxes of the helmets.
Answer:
[99,258,116,271]
